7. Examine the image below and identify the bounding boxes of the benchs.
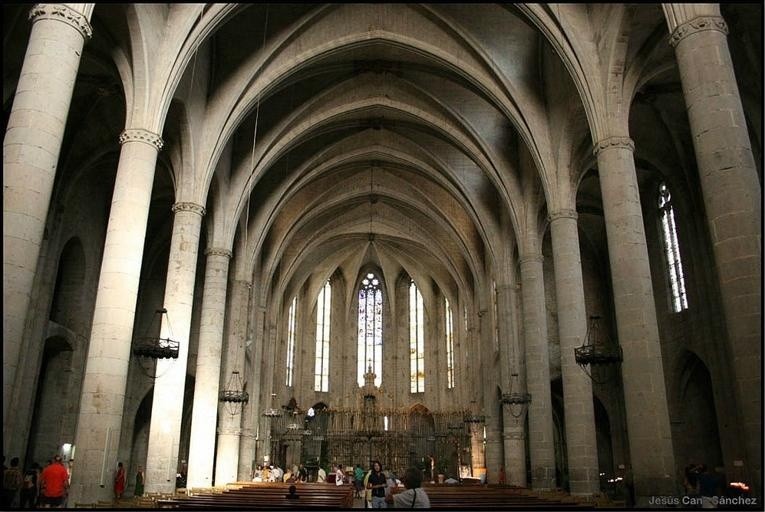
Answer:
[73,481,355,509]
[389,482,600,512]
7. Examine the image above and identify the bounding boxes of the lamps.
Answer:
[574,308,623,390]
[132,308,179,379]
[498,371,532,425]
[217,3,271,421]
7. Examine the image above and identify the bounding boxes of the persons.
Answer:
[2,454,73,508]
[317,464,327,482]
[335,463,346,486]
[444,472,459,484]
[175,459,187,488]
[112,462,125,500]
[367,461,388,508]
[283,485,299,499]
[133,468,145,498]
[253,461,310,484]
[353,463,364,499]
[682,457,702,496]
[383,466,431,507]
